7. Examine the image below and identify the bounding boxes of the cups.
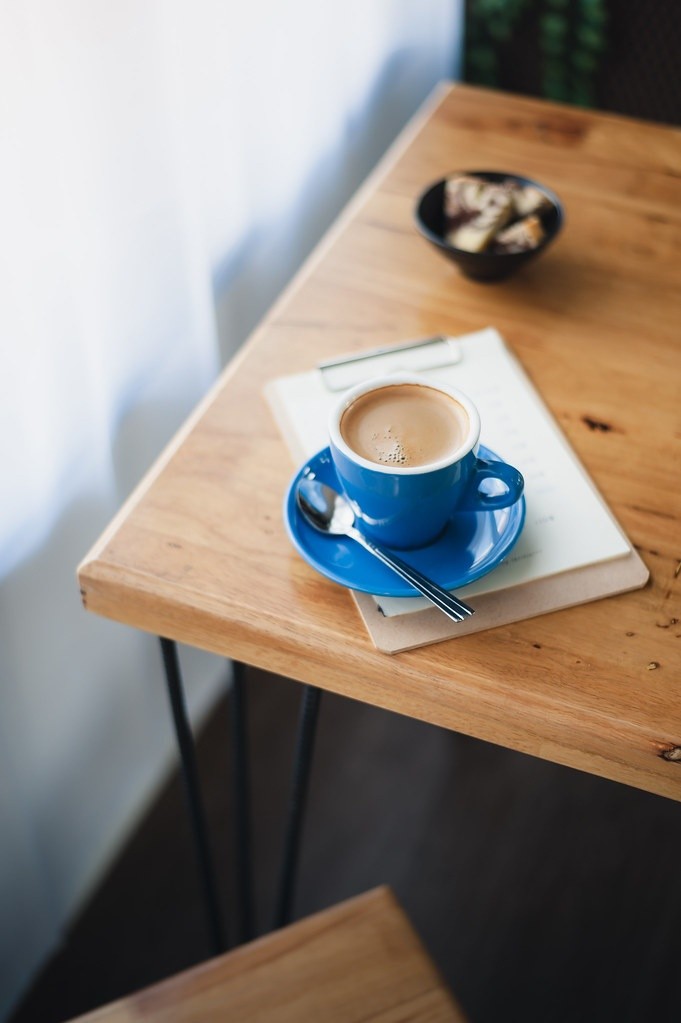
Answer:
[330,374,524,550]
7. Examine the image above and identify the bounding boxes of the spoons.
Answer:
[296,479,474,623]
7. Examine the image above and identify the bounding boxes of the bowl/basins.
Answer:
[415,172,564,284]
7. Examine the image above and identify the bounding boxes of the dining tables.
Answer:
[76,79,680,958]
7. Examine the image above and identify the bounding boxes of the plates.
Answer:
[286,442,526,598]
